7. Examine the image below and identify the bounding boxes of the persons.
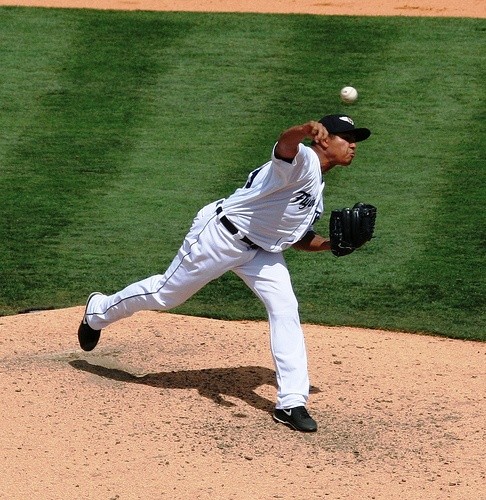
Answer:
[78,114,377,433]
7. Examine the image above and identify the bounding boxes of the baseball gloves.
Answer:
[328,201,377,257]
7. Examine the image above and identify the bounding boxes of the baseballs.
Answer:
[340,85,359,104]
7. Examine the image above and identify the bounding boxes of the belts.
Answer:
[217,201,262,249]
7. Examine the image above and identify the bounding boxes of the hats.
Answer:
[318,114,371,142]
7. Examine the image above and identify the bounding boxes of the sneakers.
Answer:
[78,292,102,351]
[273,406,318,432]
